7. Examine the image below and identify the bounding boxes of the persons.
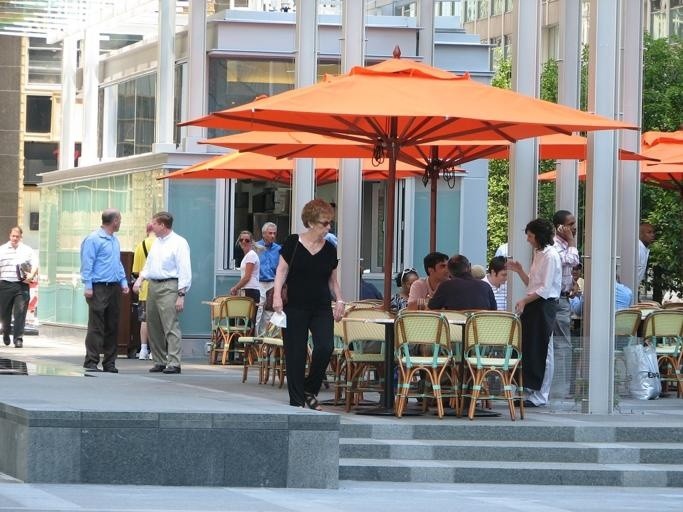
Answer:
[274,199,345,411]
[0,225,39,348]
[80,208,130,371]
[360,266,383,301]
[131,211,192,374]
[231,231,261,362]
[570,262,634,311]
[132,224,156,360]
[482,256,507,311]
[255,222,280,338]
[505,210,580,407]
[390,252,497,409]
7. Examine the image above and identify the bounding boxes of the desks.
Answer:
[356,317,502,418]
[617,306,662,321]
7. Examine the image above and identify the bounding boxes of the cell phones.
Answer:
[557,225,564,232]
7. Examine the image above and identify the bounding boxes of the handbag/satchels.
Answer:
[264,285,288,310]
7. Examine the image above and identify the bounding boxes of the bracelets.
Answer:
[338,301,344,303]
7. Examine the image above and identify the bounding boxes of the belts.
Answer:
[152,277,179,282]
[561,291,570,297]
[93,282,118,286]
[259,280,273,282]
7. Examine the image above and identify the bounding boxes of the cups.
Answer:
[416,298,426,311]
[237,290,245,297]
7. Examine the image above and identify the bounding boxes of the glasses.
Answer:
[317,220,334,227]
[239,239,250,243]
[400,269,417,283]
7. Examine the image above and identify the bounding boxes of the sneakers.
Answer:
[15,337,23,348]
[514,400,536,407]
[4,334,10,345]
[163,366,180,373]
[138,351,153,359]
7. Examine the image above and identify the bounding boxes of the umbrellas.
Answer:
[198,134,661,254]
[539,129,682,228]
[178,46,642,312]
[157,158,468,185]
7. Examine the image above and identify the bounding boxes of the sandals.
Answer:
[305,391,322,411]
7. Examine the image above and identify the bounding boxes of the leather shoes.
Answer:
[103,367,118,373]
[149,365,166,372]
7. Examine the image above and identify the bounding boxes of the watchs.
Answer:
[178,292,185,296]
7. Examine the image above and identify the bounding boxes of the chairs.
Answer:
[340,307,395,412]
[330,301,385,407]
[661,303,682,311]
[457,310,525,421]
[615,309,643,338]
[257,310,282,388]
[213,297,256,365]
[631,300,661,308]
[277,296,330,391]
[209,296,229,363]
[238,329,265,384]
[641,310,683,398]
[392,310,461,419]
